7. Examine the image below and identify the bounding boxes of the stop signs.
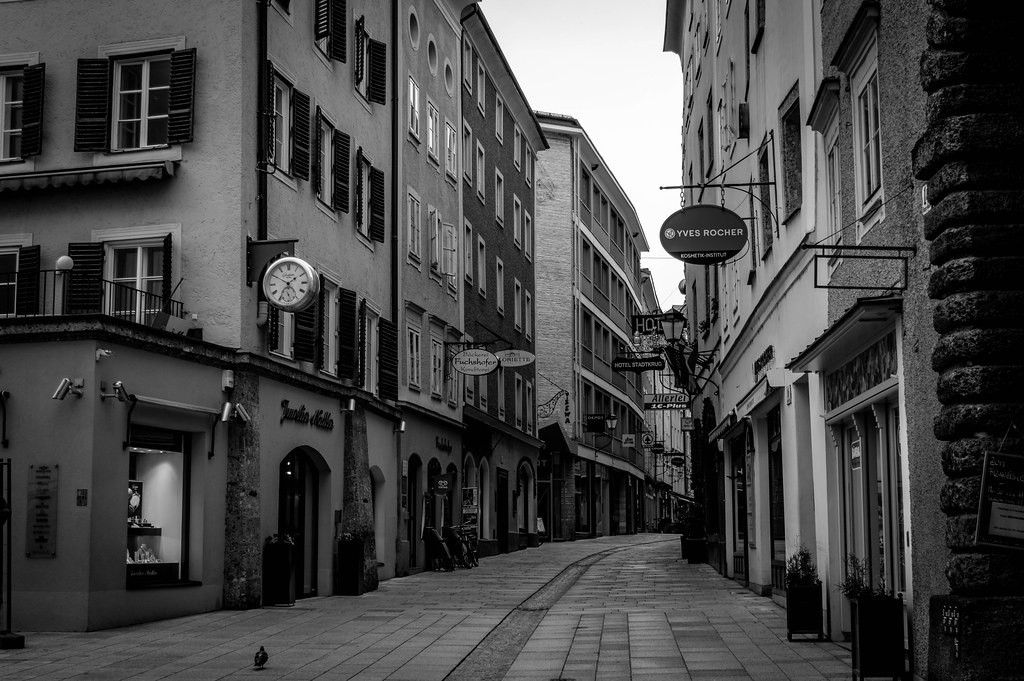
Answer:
[651,444,664,454]
[672,457,684,466]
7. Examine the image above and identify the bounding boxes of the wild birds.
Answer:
[254,646,268,670]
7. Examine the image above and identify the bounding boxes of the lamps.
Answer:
[658,304,720,359]
[595,411,618,458]
[341,398,355,413]
[218,398,252,425]
[393,419,407,433]
[49,377,130,404]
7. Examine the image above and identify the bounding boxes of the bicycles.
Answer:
[450,524,479,569]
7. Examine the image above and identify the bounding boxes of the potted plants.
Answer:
[784,543,823,641]
[832,550,905,680]
[335,531,365,596]
[262,533,296,607]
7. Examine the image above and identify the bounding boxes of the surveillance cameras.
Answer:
[236,403,251,421]
[112,381,130,402]
[222,402,232,422]
[52,378,71,400]
[400,421,405,433]
[348,399,356,412]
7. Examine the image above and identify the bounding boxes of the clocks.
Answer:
[263,256,321,313]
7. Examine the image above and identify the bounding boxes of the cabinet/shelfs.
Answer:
[126,478,179,586]
